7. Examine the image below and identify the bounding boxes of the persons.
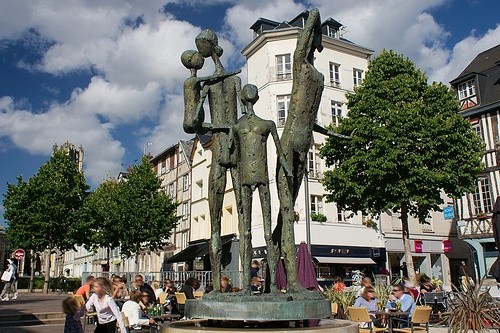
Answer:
[33,253,42,272]
[352,277,388,333]
[0,259,19,301]
[250,260,262,291]
[381,274,419,328]
[329,276,346,293]
[399,262,407,275]
[220,277,233,293]
[61,274,177,333]
[420,275,443,318]
[177,277,201,327]
[379,262,390,276]
[181,7,357,298]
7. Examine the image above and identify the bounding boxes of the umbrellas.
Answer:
[296,240,319,327]
[277,259,286,290]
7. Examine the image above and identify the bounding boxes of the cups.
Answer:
[377,300,382,310]
[397,301,401,310]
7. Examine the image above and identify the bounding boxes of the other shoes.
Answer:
[12,296,17,299]
[2,297,9,301]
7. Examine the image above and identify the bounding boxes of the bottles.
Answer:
[148,303,165,316]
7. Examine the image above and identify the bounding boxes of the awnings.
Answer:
[313,257,376,264]
[92,260,108,266]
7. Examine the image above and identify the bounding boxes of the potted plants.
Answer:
[441,265,500,333]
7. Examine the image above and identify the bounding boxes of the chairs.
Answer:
[68,287,239,333]
[331,290,450,333]
[371,271,401,323]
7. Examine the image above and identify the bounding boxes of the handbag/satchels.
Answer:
[1,268,13,282]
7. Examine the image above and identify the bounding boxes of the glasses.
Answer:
[393,290,399,292]
[369,291,374,292]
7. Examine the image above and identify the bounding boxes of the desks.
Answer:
[375,312,411,333]
[114,299,127,304]
[152,315,181,325]
